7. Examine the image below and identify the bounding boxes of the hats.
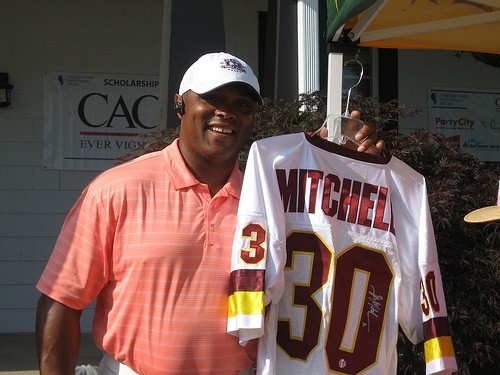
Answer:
[178,51,265,106]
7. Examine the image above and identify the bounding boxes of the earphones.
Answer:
[174,101,185,116]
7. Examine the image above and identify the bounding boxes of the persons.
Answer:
[37,51,385,375]
